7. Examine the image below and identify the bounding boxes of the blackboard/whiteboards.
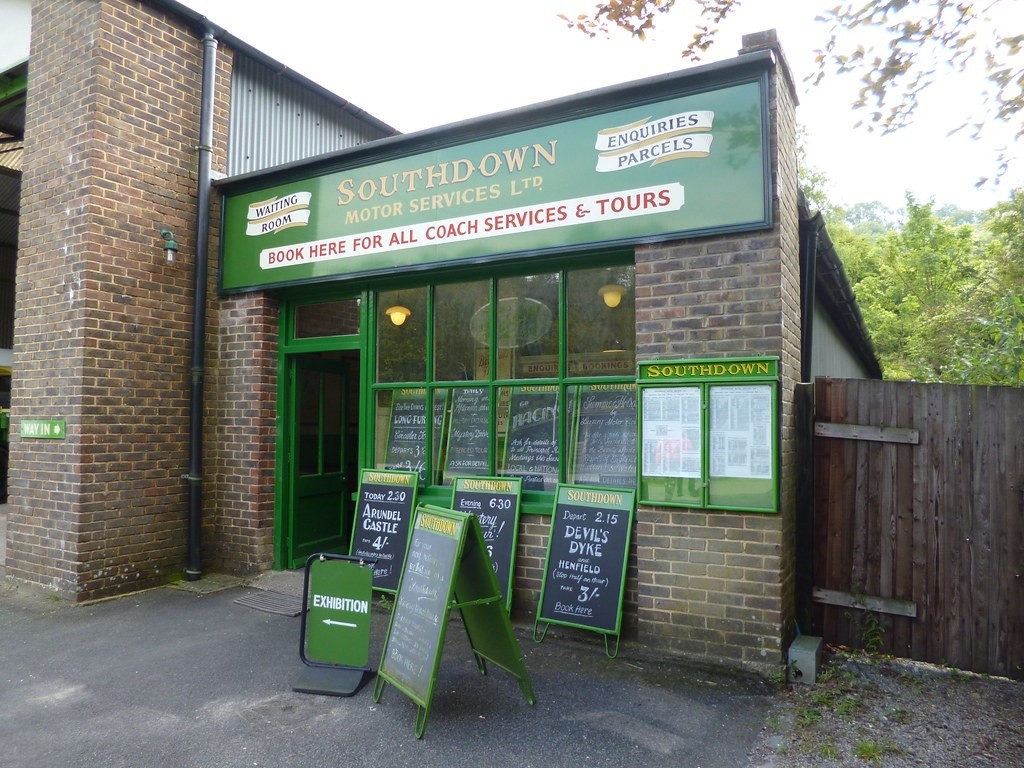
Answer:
[443,381,502,483]
[348,468,420,593]
[536,483,635,635]
[568,371,639,491]
[503,375,579,491]
[450,473,524,617]
[382,385,451,485]
[377,500,529,708]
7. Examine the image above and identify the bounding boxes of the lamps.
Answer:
[598,276,628,308]
[385,293,411,326]
[161,229,179,266]
